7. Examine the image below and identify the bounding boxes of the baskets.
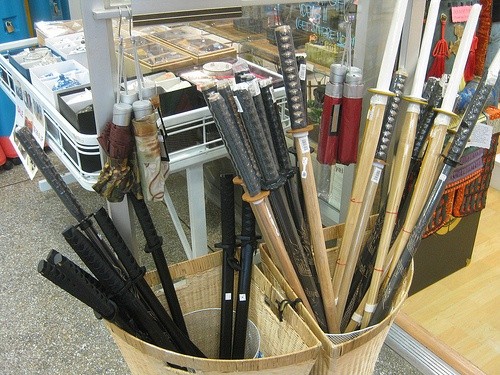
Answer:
[103,216,417,375]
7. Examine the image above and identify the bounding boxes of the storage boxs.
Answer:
[0,10,496,373]
